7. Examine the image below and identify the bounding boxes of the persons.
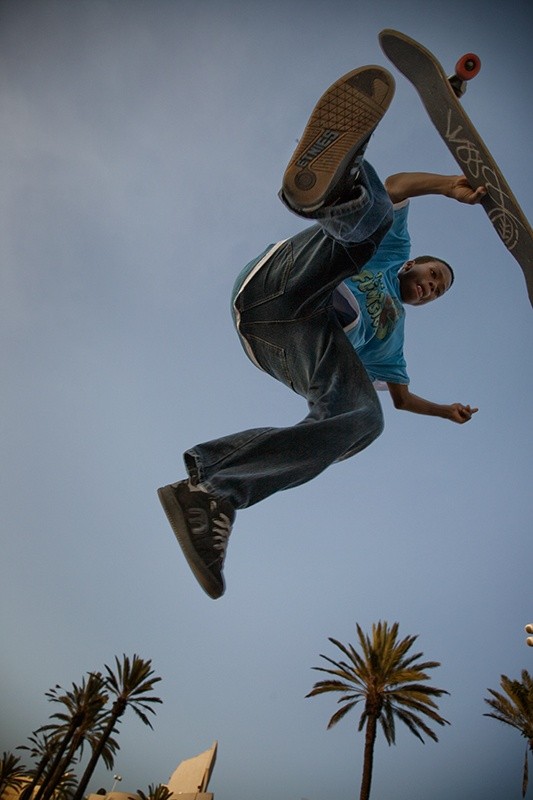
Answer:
[156,64,488,601]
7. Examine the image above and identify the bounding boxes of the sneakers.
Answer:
[280,64,396,220]
[157,480,237,599]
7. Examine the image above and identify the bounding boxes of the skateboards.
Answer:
[376,29,533,305]
